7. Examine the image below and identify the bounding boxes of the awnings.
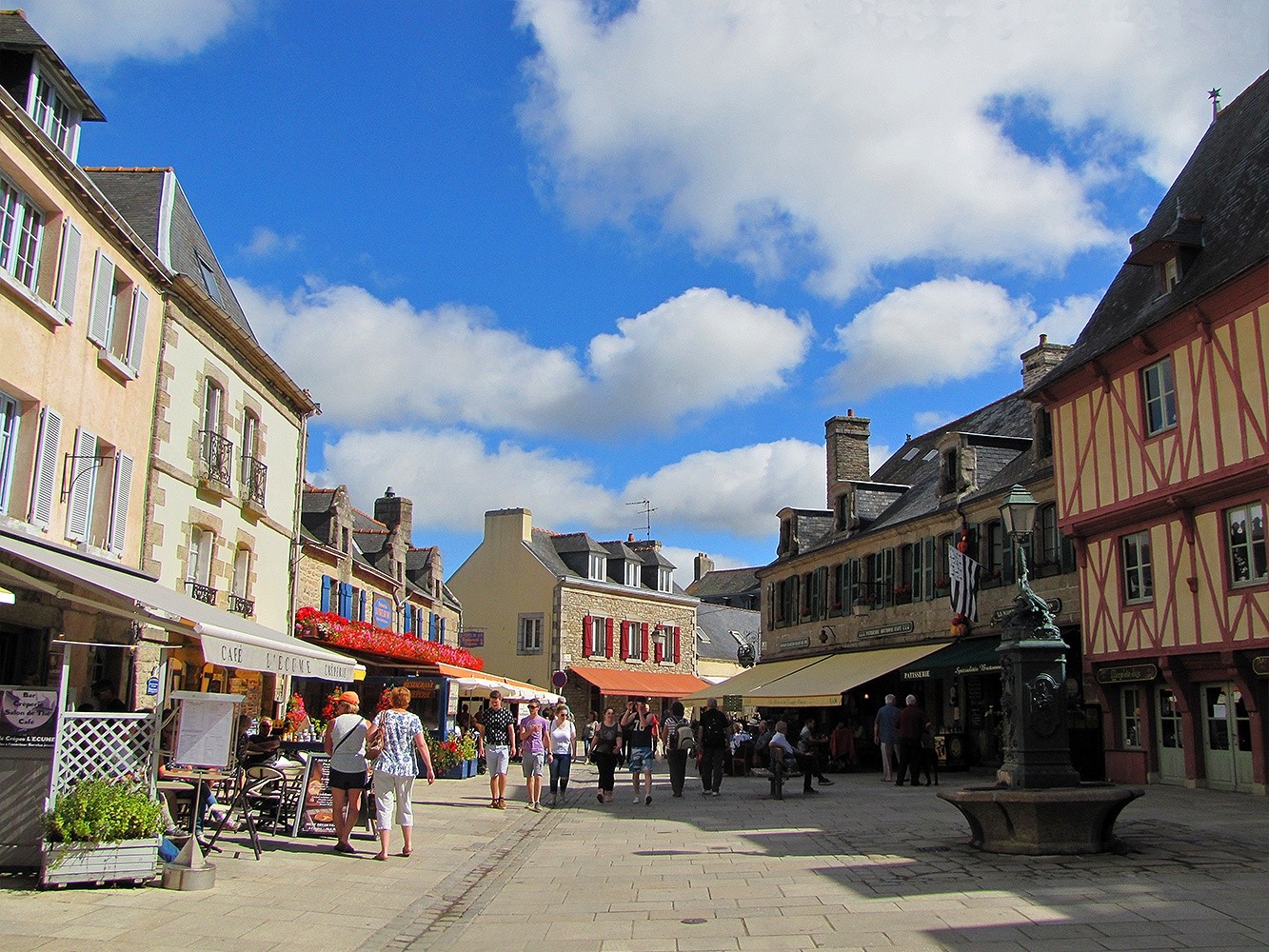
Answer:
[677,641,954,709]
[570,665,712,697]
[1,533,355,683]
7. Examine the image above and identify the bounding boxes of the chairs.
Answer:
[732,746,748,776]
[830,728,855,770]
[161,747,311,859]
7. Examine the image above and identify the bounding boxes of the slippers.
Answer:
[402,847,413,857]
[372,853,388,861]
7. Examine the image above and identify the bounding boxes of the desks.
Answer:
[813,739,838,772]
[160,774,228,833]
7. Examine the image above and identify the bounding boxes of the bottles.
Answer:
[283,724,316,742]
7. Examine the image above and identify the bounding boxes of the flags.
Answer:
[947,545,979,623]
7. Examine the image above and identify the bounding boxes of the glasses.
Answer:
[527,705,539,710]
[606,713,614,715]
[559,714,568,717]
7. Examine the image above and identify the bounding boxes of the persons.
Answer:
[72,683,434,863]
[459,694,936,813]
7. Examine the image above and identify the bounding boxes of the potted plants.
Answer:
[38,786,162,888]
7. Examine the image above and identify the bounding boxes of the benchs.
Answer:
[750,740,802,797]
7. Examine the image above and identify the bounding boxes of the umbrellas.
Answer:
[454,675,564,735]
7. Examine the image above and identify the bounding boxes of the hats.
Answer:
[334,692,359,705]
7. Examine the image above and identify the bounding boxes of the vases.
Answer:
[468,758,478,776]
[416,758,469,779]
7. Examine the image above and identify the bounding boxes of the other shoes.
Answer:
[881,777,924,786]
[524,793,569,810]
[702,788,711,795]
[818,780,834,785]
[632,796,638,803]
[597,788,604,802]
[712,791,720,796]
[644,793,653,807]
[498,798,504,808]
[335,841,355,853]
[489,799,498,808]
[805,788,819,793]
[605,792,613,801]
[216,819,241,830]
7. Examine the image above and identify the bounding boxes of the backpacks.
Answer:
[670,716,693,750]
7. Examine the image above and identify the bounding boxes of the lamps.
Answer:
[851,583,899,616]
[650,621,667,644]
[819,625,837,645]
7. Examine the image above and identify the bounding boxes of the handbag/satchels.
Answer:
[655,738,664,764]
[581,736,587,741]
[364,710,386,761]
[544,732,551,748]
[589,748,598,762]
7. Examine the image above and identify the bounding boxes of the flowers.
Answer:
[280,605,506,779]
